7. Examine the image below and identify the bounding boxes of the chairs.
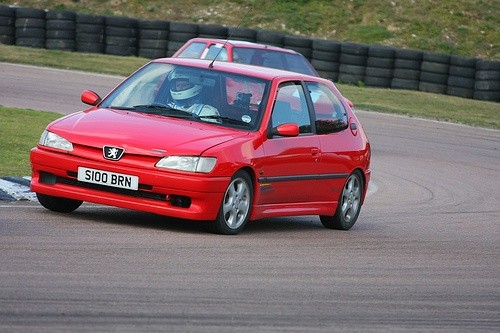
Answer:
[269,100,297,128]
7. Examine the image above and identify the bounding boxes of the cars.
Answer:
[154,38,353,126]
[29,57,371,235]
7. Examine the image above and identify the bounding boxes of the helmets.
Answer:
[169,66,205,106]
[220,49,240,63]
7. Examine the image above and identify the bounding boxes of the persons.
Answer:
[166,66,222,125]
